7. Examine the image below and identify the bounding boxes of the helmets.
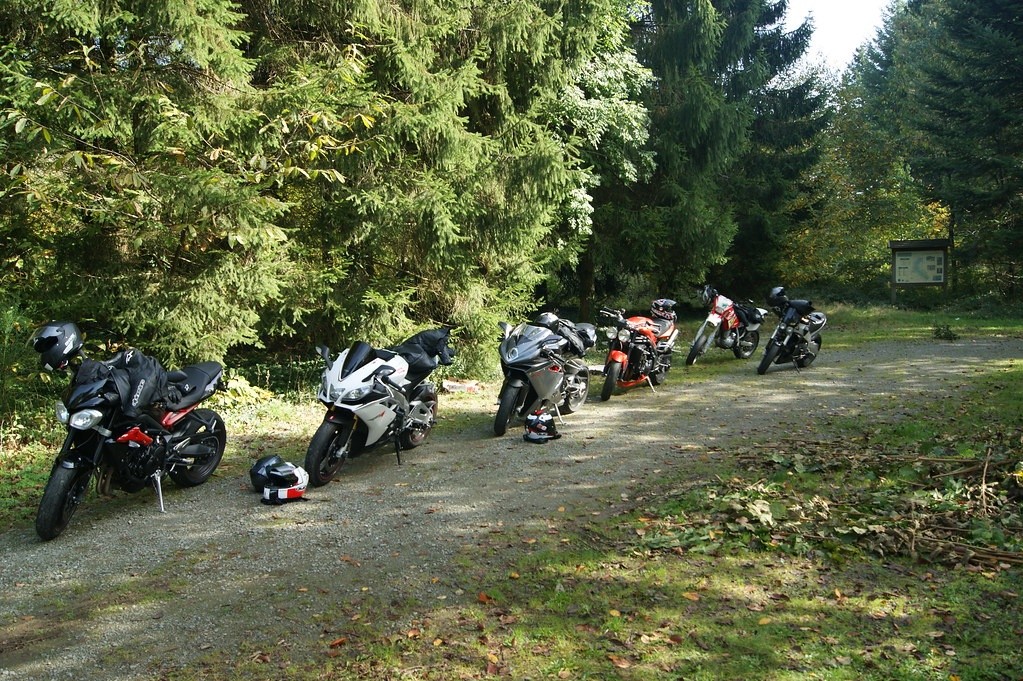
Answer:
[771,287,786,298]
[697,285,711,306]
[523,413,562,443]
[250,454,286,492]
[261,461,309,504]
[650,299,677,319]
[534,312,559,334]
[33,322,84,371]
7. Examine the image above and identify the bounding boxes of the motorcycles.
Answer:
[32,348,226,543]
[757,295,826,375]
[596,300,680,402]
[492,312,597,437]
[304,338,439,486]
[685,289,768,366]
